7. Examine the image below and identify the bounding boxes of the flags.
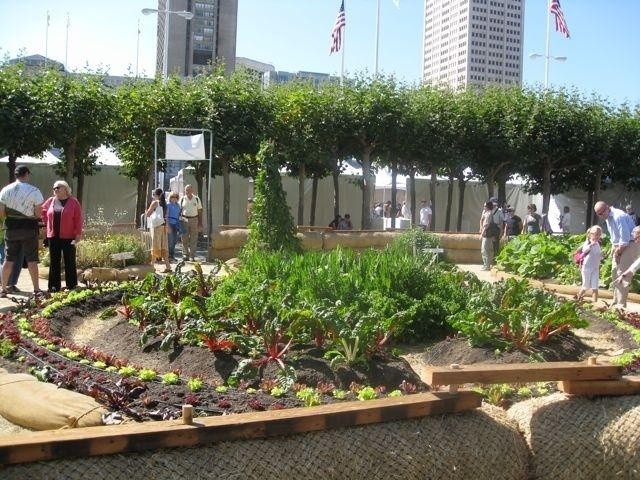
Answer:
[550,0,571,39]
[328,2,345,55]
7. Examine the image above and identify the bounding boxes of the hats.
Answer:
[490,197,538,212]
[14,165,34,178]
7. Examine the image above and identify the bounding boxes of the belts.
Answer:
[182,215,198,218]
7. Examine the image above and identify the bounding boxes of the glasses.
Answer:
[52,184,64,192]
[595,209,607,216]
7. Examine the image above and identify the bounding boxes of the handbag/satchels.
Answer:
[145,199,164,229]
[178,220,187,236]
[482,223,499,238]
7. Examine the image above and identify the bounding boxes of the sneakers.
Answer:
[0,281,76,300]
[153,257,201,274]
[480,265,491,273]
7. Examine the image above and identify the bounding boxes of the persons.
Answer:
[0,215,25,293]
[576,225,602,302]
[144,184,203,273]
[340,214,353,230]
[561,206,570,232]
[0,164,45,299]
[40,179,83,294]
[418,199,432,231]
[374,200,408,219]
[593,201,636,310]
[630,225,640,244]
[479,196,543,271]
[626,204,640,226]
[328,214,344,230]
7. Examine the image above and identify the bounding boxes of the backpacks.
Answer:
[573,241,601,265]
